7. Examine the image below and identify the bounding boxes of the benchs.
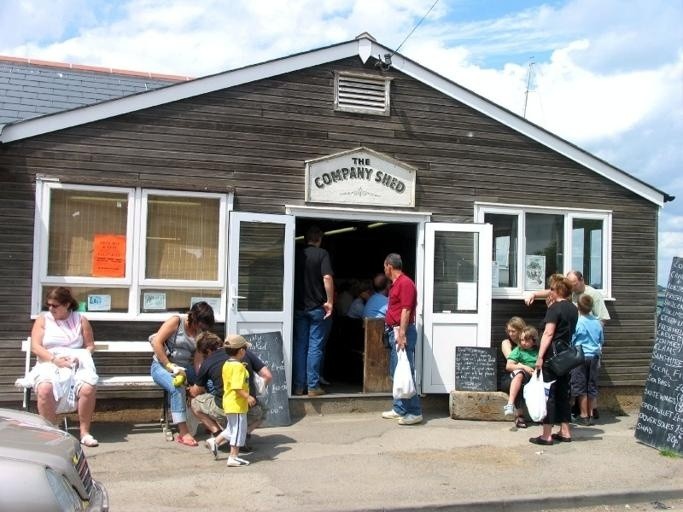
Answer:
[14,336,172,432]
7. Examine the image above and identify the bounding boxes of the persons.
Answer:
[150,300,214,446]
[500,270,611,445]
[292,224,333,395]
[28,287,101,446]
[189,331,271,451]
[379,253,423,425]
[205,334,257,466]
[316,273,391,389]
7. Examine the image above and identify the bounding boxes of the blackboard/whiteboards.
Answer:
[455,346,498,391]
[242,332,292,428]
[634,257,683,457]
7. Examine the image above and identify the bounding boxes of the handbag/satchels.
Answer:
[146,332,172,361]
[382,322,393,350]
[545,338,586,376]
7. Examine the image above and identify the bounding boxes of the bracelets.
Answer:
[50,356,54,362]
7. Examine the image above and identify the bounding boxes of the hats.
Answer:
[223,334,252,350]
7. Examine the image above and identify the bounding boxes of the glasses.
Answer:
[45,301,64,308]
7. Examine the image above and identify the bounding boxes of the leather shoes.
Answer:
[320,377,331,386]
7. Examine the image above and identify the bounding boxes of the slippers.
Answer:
[79,434,99,447]
[551,433,572,442]
[528,435,554,446]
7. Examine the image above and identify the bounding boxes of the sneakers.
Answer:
[571,413,594,426]
[381,408,402,419]
[295,388,303,395]
[306,387,326,397]
[593,408,599,419]
[504,404,514,416]
[205,429,251,460]
[225,455,250,468]
[398,413,423,425]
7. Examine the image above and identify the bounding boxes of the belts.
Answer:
[392,323,415,328]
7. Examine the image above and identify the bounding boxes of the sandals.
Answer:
[514,414,527,428]
[176,433,198,446]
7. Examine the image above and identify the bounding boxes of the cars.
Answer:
[1,405,110,512]
